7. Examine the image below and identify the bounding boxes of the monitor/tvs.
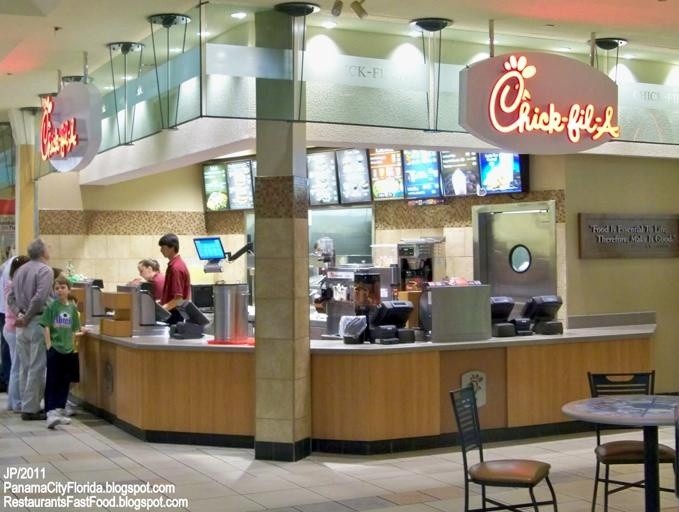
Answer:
[193,237,226,260]
[176,300,211,326]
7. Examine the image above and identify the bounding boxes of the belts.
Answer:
[18,310,44,317]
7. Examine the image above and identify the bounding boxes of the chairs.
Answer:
[588,369,679,512]
[446,382,559,512]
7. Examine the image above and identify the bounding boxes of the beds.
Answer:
[560,394,679,512]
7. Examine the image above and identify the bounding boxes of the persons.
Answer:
[159,233,191,322]
[7,239,54,422]
[37,278,82,429]
[0,256,20,395]
[3,256,31,414]
[138,260,165,302]
[65,295,88,416]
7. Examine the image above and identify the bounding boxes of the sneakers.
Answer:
[45,407,63,430]
[56,407,72,425]
[22,411,47,420]
